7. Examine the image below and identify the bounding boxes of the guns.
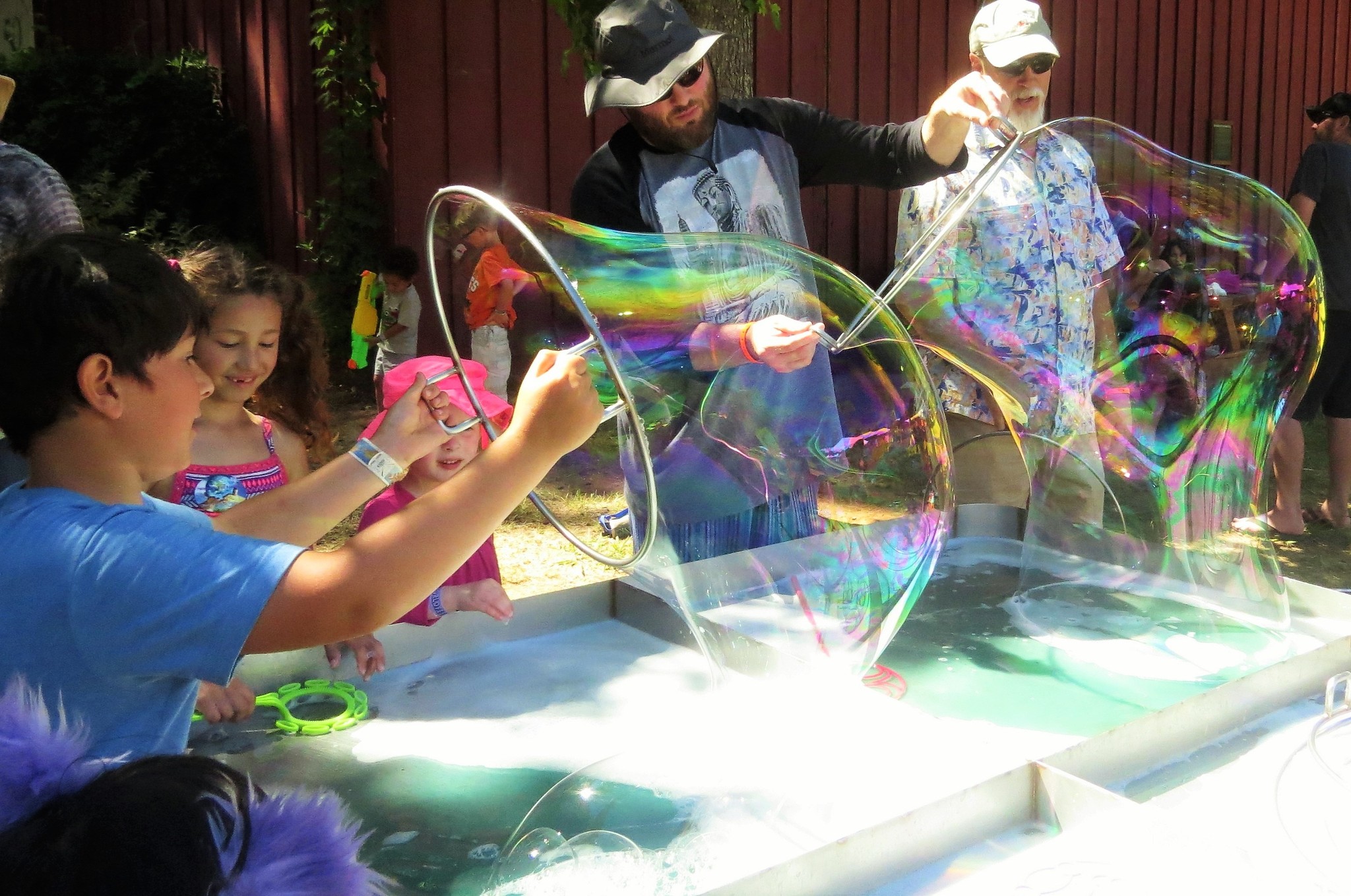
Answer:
[347,269,386,371]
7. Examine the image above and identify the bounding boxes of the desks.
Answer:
[1206,287,1258,351]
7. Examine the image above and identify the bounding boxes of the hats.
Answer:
[969,0,1060,68]
[584,0,739,117]
[357,356,513,449]
[1306,92,1351,123]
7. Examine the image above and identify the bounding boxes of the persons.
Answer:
[551,0,1010,575]
[364,246,421,407]
[0,757,378,896]
[148,239,387,725]
[359,358,514,628]
[897,0,1131,614]
[1232,88,1351,535]
[0,232,604,833]
[453,202,531,417]
[1136,238,1210,441]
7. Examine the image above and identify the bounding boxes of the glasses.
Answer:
[976,53,1053,79]
[655,58,704,102]
[461,226,487,242]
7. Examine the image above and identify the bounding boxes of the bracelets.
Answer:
[348,437,404,487]
[739,320,765,364]
[428,587,447,617]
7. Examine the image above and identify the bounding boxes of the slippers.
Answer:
[1300,504,1333,528]
[1231,516,1307,540]
